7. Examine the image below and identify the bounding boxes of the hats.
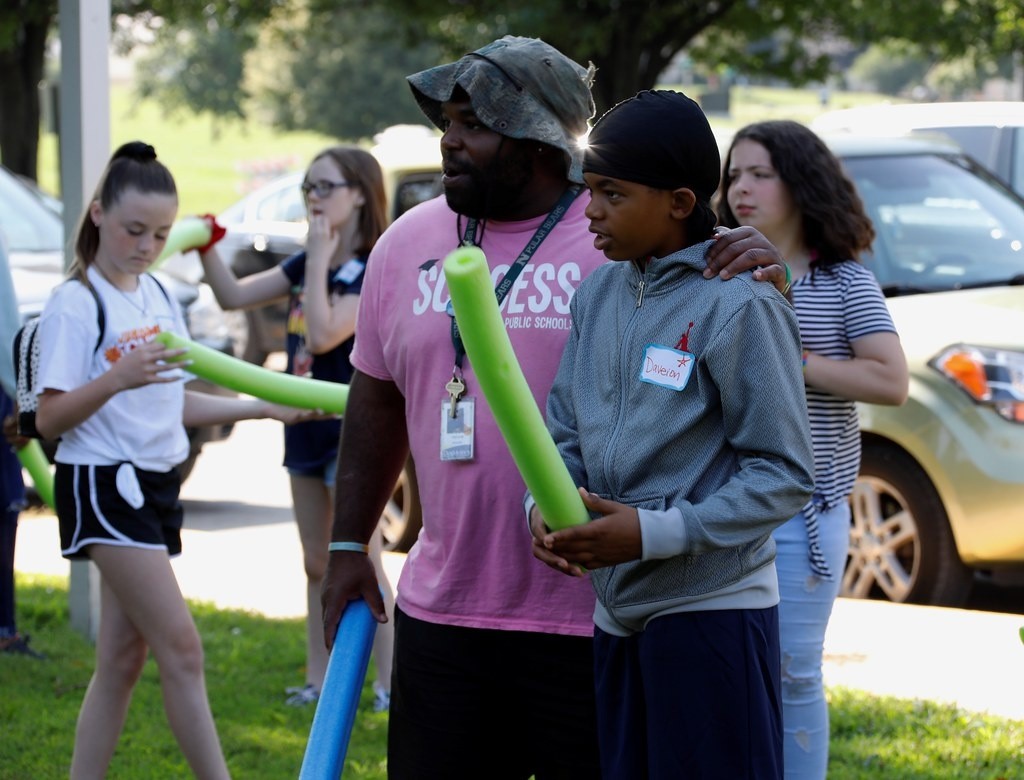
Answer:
[405,35,597,184]
[582,91,720,205]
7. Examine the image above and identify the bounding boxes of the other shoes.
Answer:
[285,684,319,707]
[373,684,390,711]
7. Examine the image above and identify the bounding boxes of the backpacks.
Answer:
[13,275,170,439]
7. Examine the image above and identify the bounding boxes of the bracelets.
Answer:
[803,350,809,379]
[328,541,370,553]
[783,259,792,295]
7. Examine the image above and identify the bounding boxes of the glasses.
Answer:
[302,180,349,199]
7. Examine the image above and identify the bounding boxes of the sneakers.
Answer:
[0,626,45,660]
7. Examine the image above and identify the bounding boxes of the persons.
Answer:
[323,34,792,780]
[0,243,30,650]
[716,121,909,780]
[187,148,394,711]
[524,91,815,780]
[37,141,337,780]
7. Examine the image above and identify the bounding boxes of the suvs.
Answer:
[0,165,243,506]
[811,98,1024,202]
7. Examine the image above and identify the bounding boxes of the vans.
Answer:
[358,123,1024,609]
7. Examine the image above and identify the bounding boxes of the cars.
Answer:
[177,165,310,354]
